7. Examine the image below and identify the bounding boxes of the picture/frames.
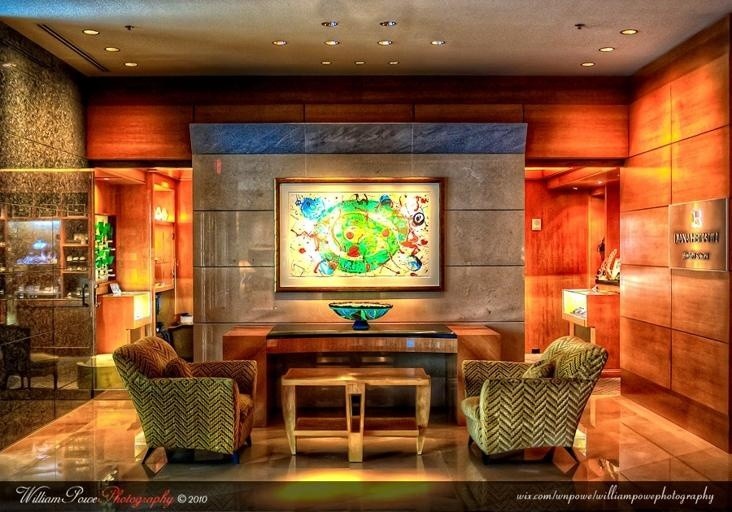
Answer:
[273,176,445,293]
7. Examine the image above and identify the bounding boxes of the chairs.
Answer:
[461,335,608,467]
[113,336,258,464]
[0,324,59,392]
[77,353,126,389]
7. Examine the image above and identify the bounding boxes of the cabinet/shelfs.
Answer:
[61,216,93,298]
[152,182,177,335]
[1,219,11,300]
[96,291,152,356]
[562,288,621,376]
[17,298,93,355]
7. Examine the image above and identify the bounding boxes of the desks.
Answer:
[222,323,503,427]
